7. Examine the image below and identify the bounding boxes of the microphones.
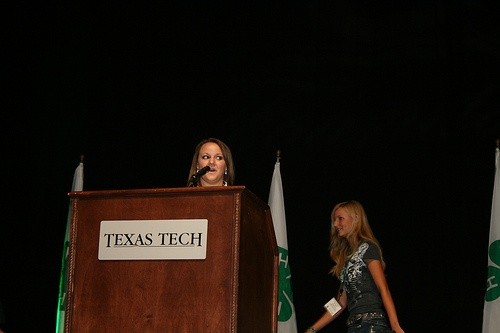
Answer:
[192,166,210,180]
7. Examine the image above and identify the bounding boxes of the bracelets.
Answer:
[307,327,318,333]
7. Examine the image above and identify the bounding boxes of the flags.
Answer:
[266,150,297,333]
[55,156,81,333]
[481,149,499,333]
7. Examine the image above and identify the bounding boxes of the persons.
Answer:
[187,139,235,187]
[304,200,404,333]
